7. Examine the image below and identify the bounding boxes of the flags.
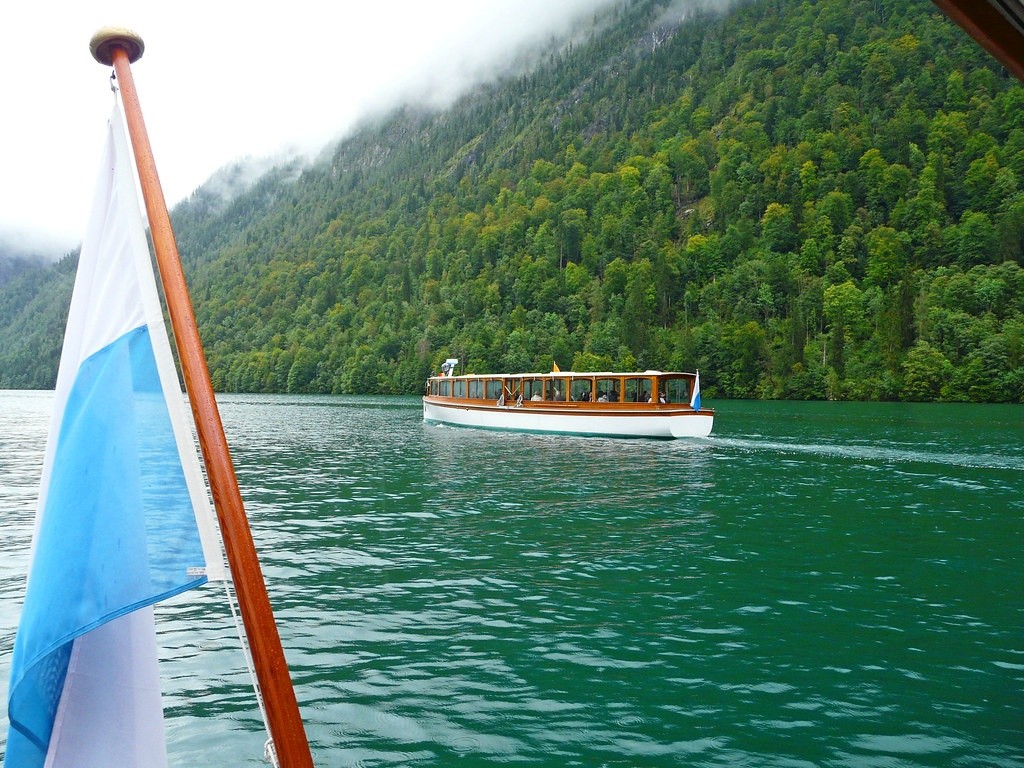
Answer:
[554,364,560,372]
[690,373,701,413]
[3,103,225,768]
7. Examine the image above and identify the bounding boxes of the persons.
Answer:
[496,389,519,400]
[531,391,541,401]
[633,390,671,403]
[546,390,550,400]
[565,391,574,401]
[583,390,620,402]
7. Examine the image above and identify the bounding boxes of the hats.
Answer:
[533,391,539,394]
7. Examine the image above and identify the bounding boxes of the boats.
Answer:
[421,356,714,440]
